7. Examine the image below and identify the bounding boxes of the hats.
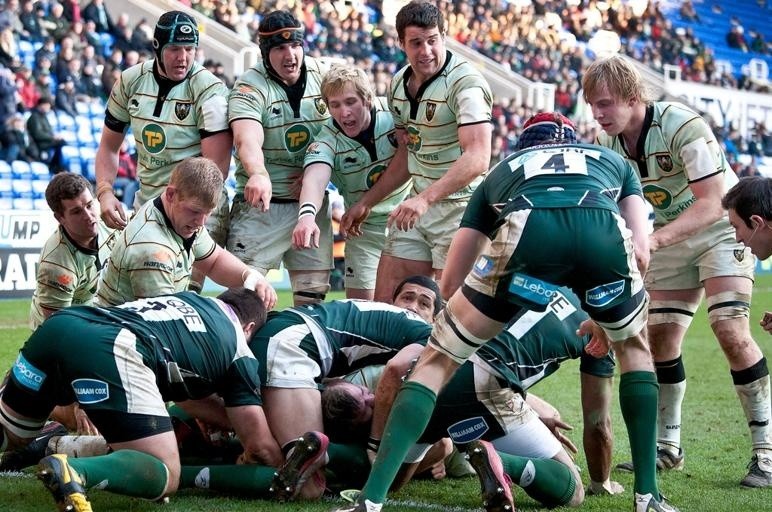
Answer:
[515,111,577,152]
[152,10,200,75]
[258,11,306,78]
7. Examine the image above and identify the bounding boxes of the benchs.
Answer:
[661,0,772,80]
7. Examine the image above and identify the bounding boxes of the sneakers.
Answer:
[268,431,329,503]
[741,454,772,489]
[34,452,92,512]
[465,440,515,512]
[0,420,69,474]
[616,445,685,474]
[633,489,680,511]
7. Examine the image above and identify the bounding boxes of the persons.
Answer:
[2,2,772,511]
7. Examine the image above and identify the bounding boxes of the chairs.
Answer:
[0,33,136,210]
